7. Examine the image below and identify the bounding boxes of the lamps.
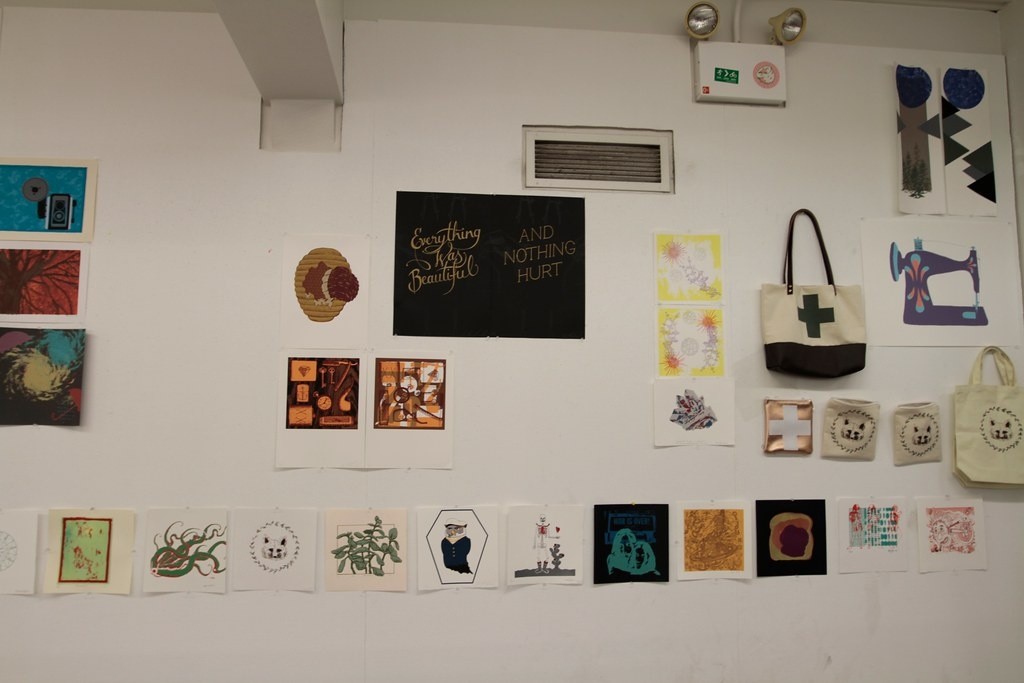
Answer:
[682,0,808,107]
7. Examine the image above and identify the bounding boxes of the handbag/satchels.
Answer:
[954,346,1024,489]
[762,209,867,380]
